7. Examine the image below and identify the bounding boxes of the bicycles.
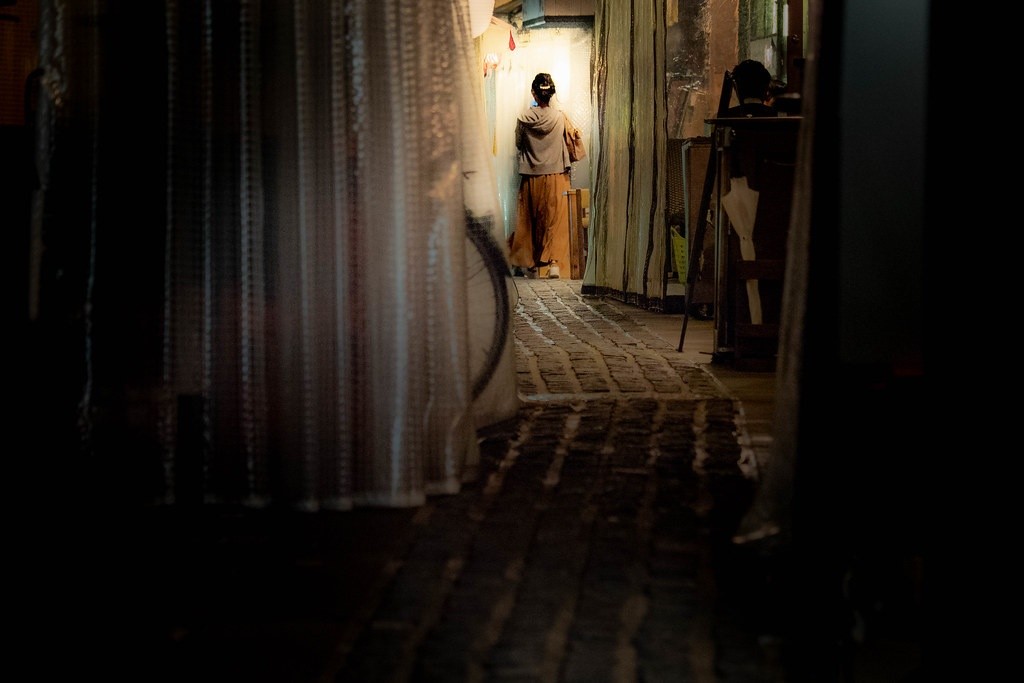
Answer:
[463,210,513,403]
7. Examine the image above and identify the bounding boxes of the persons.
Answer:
[508,73,571,278]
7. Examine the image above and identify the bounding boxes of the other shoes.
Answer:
[546,262,561,277]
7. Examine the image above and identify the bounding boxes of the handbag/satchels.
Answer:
[564,116,587,162]
